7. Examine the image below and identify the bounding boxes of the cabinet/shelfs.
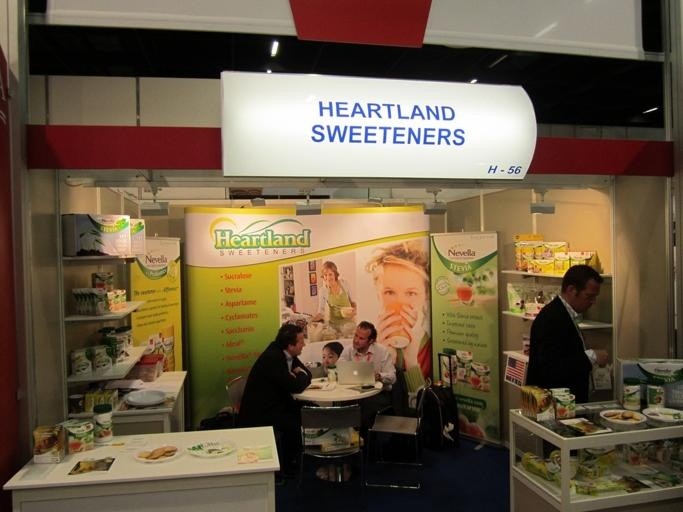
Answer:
[441,347,457,384]
[89,402,113,442]
[326,363,337,382]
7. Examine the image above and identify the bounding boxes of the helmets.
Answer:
[641,408,682,423]
[134,442,182,465]
[124,389,169,408]
[600,409,646,425]
[189,438,236,457]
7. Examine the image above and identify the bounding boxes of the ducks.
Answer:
[416,354,459,452]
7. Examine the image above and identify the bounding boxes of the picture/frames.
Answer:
[290,376,384,487]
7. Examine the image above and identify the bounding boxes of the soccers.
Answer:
[457,287,472,301]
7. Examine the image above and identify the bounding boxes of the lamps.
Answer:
[226,374,247,414]
[296,402,367,496]
[363,377,433,490]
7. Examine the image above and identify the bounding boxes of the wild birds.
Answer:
[337,360,376,385]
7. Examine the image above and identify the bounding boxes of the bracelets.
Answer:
[340,306,354,318]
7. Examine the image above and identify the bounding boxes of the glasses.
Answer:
[136,186,555,218]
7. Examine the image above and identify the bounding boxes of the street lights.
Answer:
[200,412,236,427]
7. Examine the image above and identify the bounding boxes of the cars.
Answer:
[383,300,415,349]
[69,393,84,415]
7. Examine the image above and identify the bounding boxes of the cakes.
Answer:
[646,378,665,408]
[69,395,83,413]
[442,348,457,385]
[92,404,113,442]
[622,377,641,411]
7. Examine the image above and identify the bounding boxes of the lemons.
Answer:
[306,321,323,342]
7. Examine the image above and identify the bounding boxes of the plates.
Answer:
[502,269,617,372]
[61,254,189,437]
[508,400,682,511]
[0,424,282,511]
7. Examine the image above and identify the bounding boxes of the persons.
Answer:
[236,318,310,484]
[317,341,342,376]
[336,320,397,440]
[305,261,356,336]
[362,243,434,409]
[520,263,612,461]
